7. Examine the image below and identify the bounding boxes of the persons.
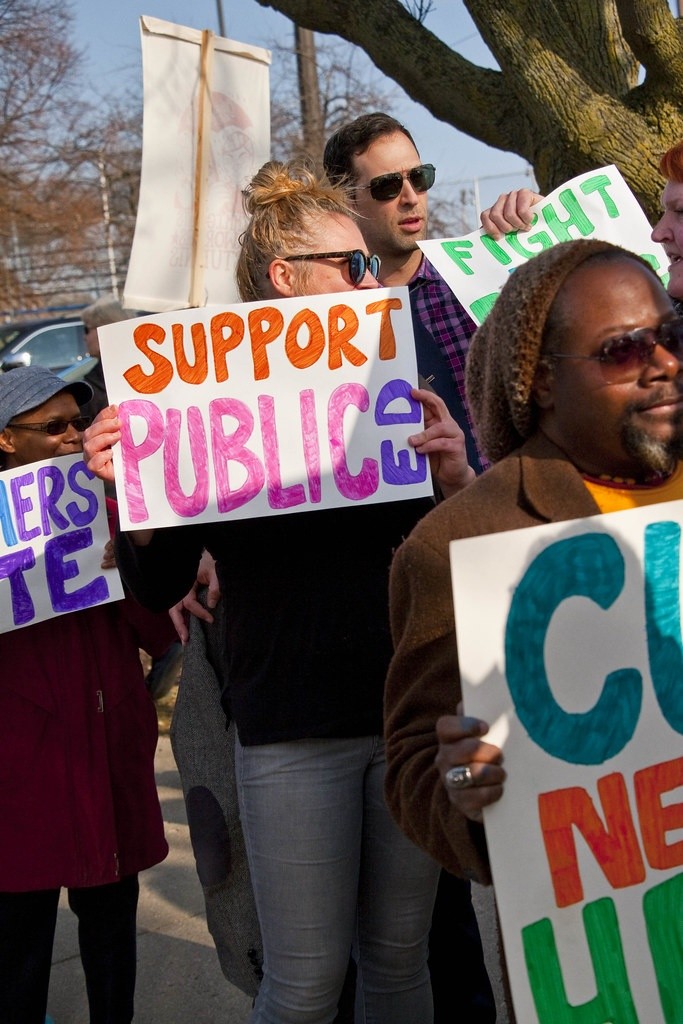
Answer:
[650,141,683,318]
[322,112,545,1024]
[0,296,218,1024]
[83,158,479,1024]
[378,238,682,1024]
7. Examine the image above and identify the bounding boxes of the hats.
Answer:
[0,365,95,432]
[463,241,665,465]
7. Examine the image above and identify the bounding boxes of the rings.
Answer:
[445,767,473,789]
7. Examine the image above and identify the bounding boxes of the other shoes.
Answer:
[145,646,185,700]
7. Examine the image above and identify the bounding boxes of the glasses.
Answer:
[265,249,382,285]
[6,416,92,435]
[534,320,683,382]
[349,164,436,201]
[83,326,96,334]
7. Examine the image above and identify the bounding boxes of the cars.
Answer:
[0,320,90,375]
